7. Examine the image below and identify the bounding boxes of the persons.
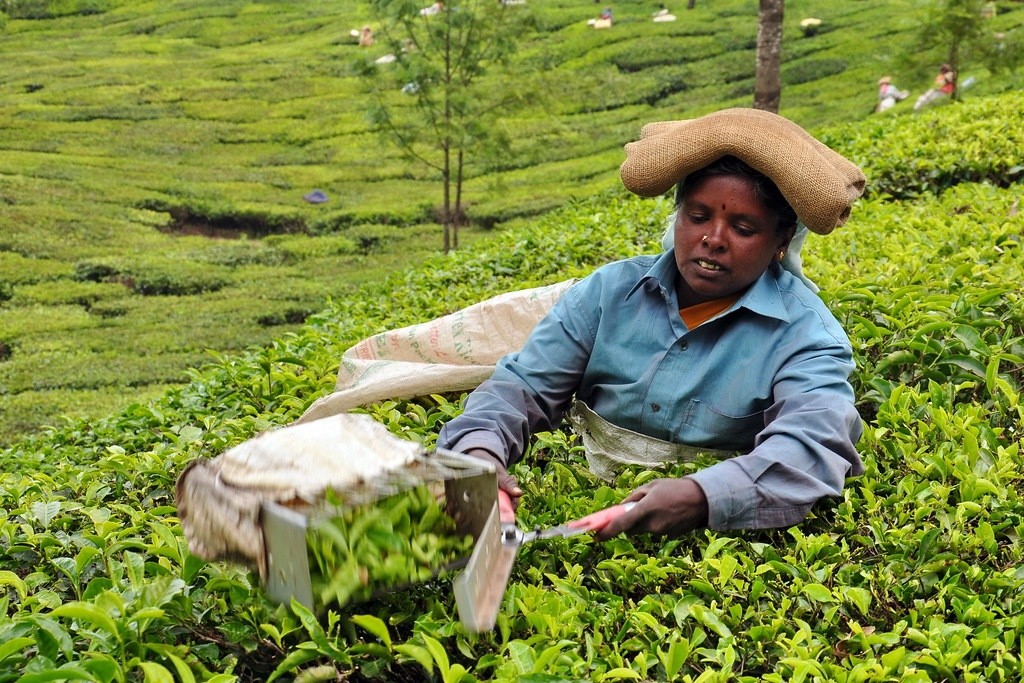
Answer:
[935,63,954,94]
[434,155,862,543]
[874,76,902,112]
[601,7,614,23]
[359,25,375,46]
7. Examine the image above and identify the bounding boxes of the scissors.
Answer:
[446,487,642,635]
